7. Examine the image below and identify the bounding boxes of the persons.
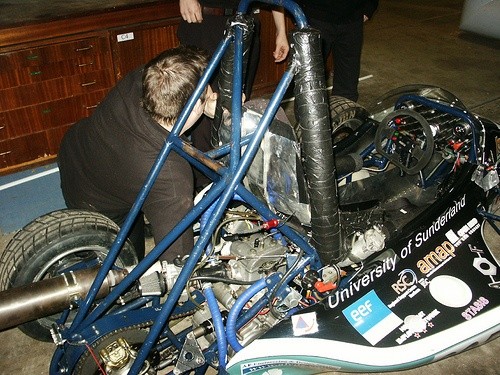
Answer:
[178,0,291,160]
[60,44,246,276]
[287,0,380,104]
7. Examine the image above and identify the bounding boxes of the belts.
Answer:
[202,6,237,16]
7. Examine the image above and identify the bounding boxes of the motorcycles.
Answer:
[0,0,500,373]
